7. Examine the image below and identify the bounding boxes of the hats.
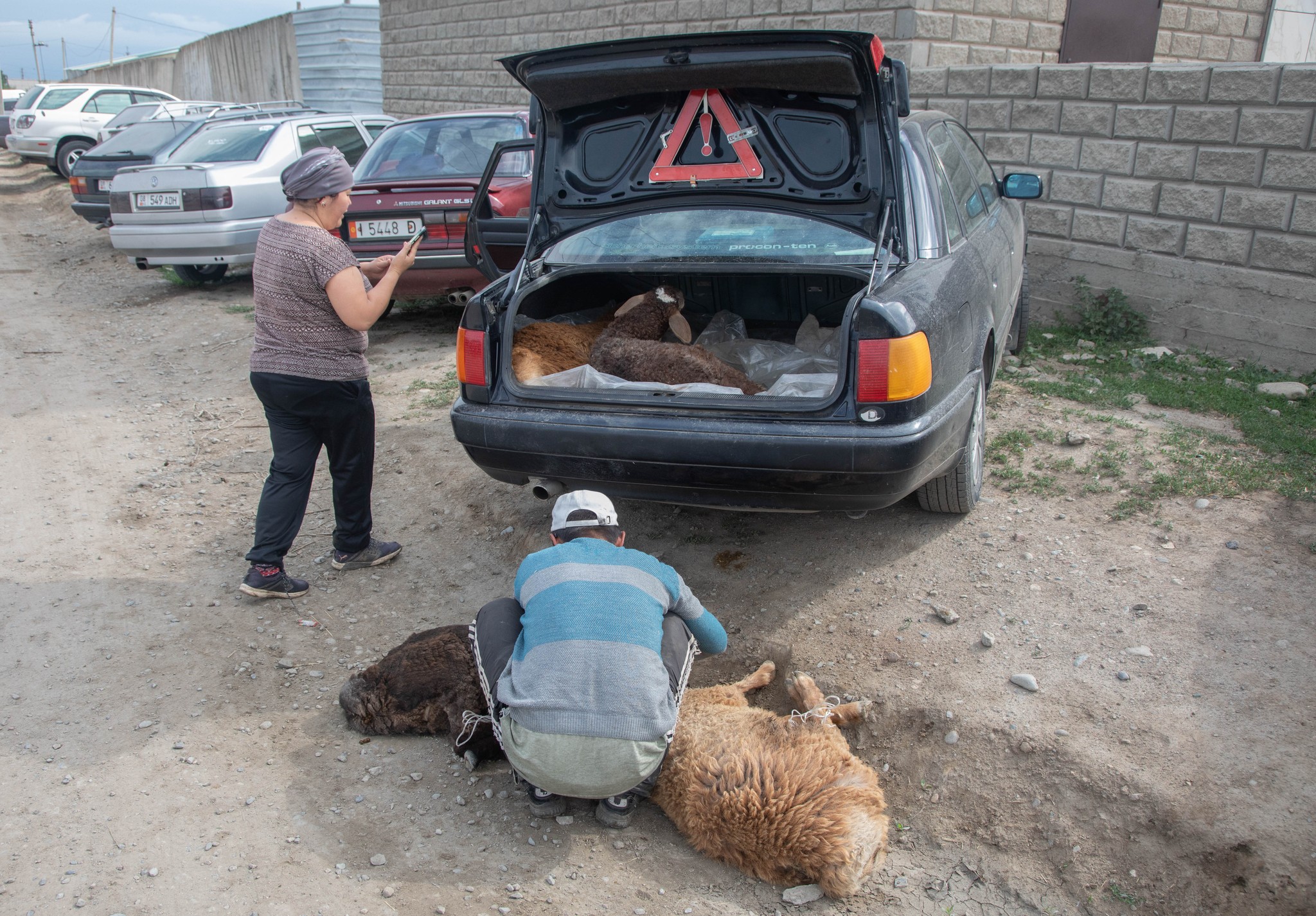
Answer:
[551,490,619,532]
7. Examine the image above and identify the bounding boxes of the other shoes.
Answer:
[520,778,566,818]
[596,790,645,830]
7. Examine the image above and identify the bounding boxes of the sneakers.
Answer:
[238,560,310,598]
[330,539,402,572]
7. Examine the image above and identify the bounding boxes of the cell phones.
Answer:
[409,226,427,245]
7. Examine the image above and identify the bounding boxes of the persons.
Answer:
[238,144,425,599]
[467,488,728,830]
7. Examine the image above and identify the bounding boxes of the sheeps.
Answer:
[333,618,507,774]
[588,283,770,398]
[511,316,616,385]
[629,657,893,901]
